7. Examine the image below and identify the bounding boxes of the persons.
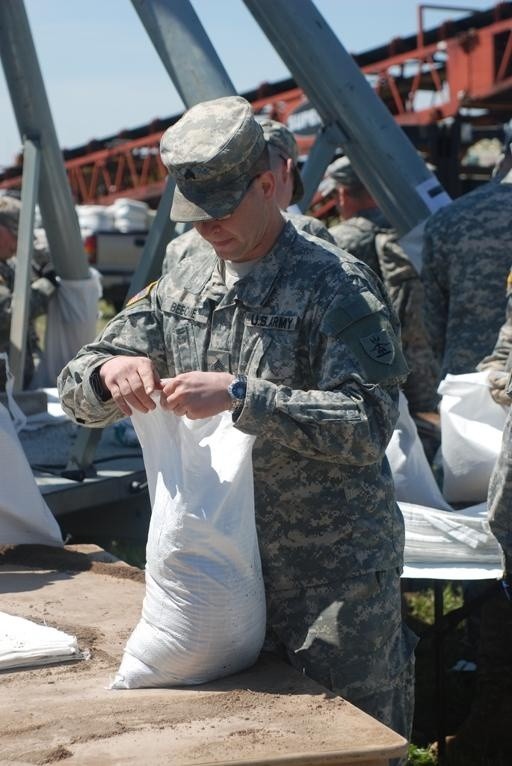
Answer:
[321,121,512,578]
[0,195,62,392]
[58,96,420,766]
[161,117,337,276]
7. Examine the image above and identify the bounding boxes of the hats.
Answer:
[259,118,305,206]
[159,95,267,224]
[328,155,365,188]
[0,196,38,241]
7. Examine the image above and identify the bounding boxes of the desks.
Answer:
[1,539,415,764]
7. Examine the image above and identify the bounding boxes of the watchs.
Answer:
[228,374,247,417]
